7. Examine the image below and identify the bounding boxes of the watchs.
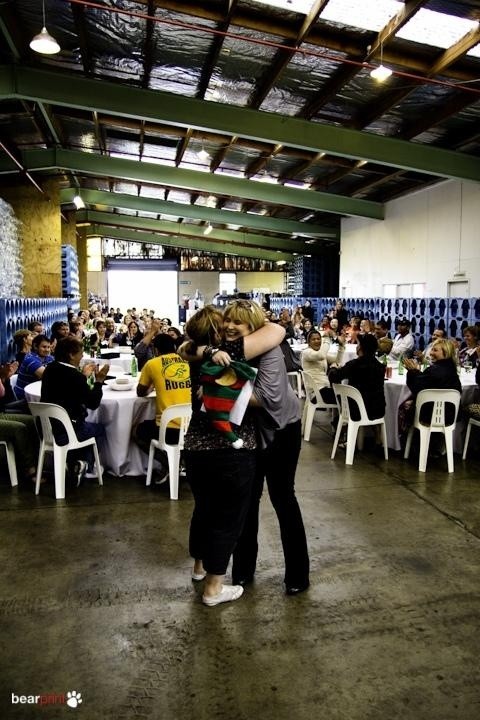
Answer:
[208,346,220,364]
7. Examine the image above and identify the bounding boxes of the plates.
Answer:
[110,383,133,390]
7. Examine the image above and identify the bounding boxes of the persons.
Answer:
[176,299,311,596]
[183,306,287,607]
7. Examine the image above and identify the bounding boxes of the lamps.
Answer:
[28,0,63,55]
[368,38,395,83]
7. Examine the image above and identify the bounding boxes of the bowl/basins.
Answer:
[116,378,130,384]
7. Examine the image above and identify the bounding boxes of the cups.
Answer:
[385,367,392,378]
[0,198,25,299]
[464,362,472,373]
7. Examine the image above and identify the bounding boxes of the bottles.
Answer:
[454,348,462,375]
[131,356,138,378]
[423,358,427,368]
[398,353,404,375]
[382,353,387,367]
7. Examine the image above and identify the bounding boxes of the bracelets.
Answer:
[203,345,215,360]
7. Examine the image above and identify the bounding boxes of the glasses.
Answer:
[228,297,255,312]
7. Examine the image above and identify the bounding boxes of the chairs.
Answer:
[0,322,480,502]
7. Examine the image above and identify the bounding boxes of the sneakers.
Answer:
[191,570,207,582]
[72,459,105,488]
[286,587,302,595]
[202,577,252,606]
[155,463,169,484]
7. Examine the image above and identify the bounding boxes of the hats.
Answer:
[356,333,378,351]
[198,360,256,451]
